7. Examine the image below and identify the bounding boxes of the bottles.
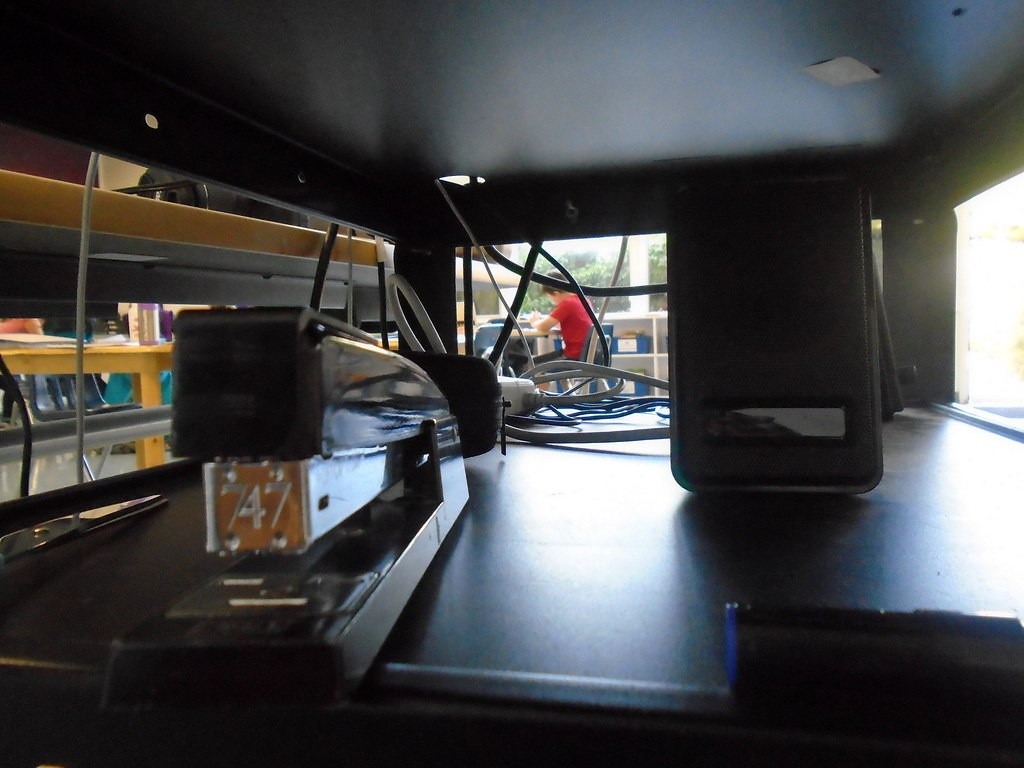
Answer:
[128,303,139,343]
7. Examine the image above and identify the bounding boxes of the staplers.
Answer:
[100,305,473,723]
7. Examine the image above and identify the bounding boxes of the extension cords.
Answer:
[497,375,536,417]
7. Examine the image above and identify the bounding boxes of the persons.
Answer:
[530,269,593,396]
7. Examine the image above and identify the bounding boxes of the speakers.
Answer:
[665,176,883,494]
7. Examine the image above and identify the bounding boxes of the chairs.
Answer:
[30,374,143,481]
[474,324,516,378]
[561,323,616,395]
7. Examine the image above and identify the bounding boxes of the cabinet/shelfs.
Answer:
[588,312,668,394]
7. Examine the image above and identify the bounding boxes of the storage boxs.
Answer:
[611,331,651,353]
[608,373,650,395]
[557,378,598,394]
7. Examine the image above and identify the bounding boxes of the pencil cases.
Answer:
[721,603,1024,743]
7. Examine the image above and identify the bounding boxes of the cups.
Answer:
[138,303,159,345]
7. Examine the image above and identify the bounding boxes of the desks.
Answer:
[0,345,174,468]
[0,167,392,496]
[508,328,560,339]
